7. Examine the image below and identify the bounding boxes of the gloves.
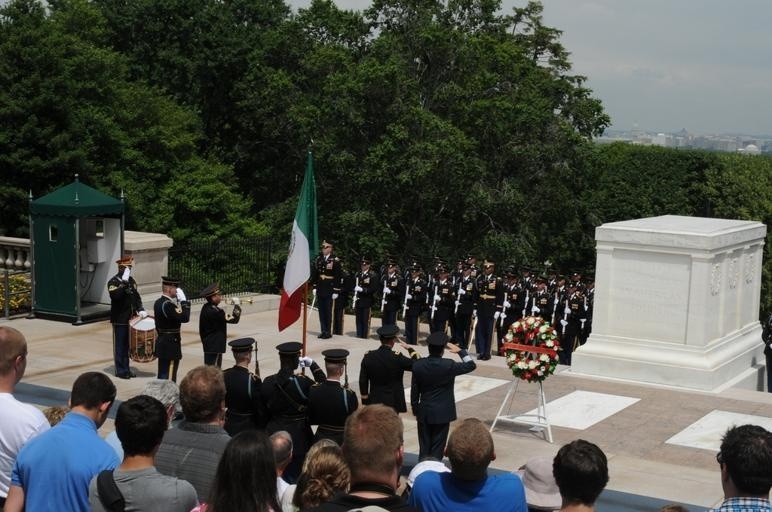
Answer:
[580,318,587,322]
[500,312,507,318]
[503,300,511,308]
[564,308,572,315]
[354,285,364,292]
[231,297,240,306]
[121,266,131,283]
[560,320,569,327]
[553,298,559,304]
[138,310,148,319]
[312,289,317,296]
[382,287,391,294]
[472,309,478,317]
[298,355,313,367]
[175,287,187,302]
[457,288,465,295]
[434,294,441,301]
[493,311,500,319]
[407,294,412,299]
[331,293,339,300]
[531,306,540,313]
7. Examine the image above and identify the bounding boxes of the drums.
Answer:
[129,314,158,363]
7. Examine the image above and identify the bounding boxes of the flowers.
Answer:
[502,316,558,382]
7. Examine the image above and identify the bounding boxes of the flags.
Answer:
[278,143,318,331]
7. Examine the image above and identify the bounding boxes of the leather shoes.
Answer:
[322,334,332,339]
[128,371,137,377]
[482,354,491,360]
[318,332,324,339]
[116,372,131,379]
[477,354,485,360]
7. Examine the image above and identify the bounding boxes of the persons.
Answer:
[452,260,465,279]
[352,260,379,338]
[377,259,405,331]
[551,274,567,297]
[270,431,293,512]
[292,446,352,512]
[262,342,326,484]
[153,365,232,506]
[546,269,558,294]
[87,395,199,512]
[0,325,51,510]
[465,254,481,278]
[518,267,532,285]
[187,431,286,511]
[427,265,452,332]
[1,372,120,511]
[549,439,608,512]
[408,418,527,512]
[493,270,526,358]
[448,264,479,347]
[400,264,427,347]
[154,276,192,384]
[311,404,415,512]
[360,324,421,416]
[222,338,263,437]
[334,254,350,335]
[526,275,555,325]
[141,379,180,429]
[568,271,588,297]
[107,258,147,379]
[313,239,344,339]
[411,332,477,462]
[429,258,448,278]
[582,277,594,338]
[552,282,586,366]
[309,349,359,447]
[474,257,504,360]
[199,282,242,365]
[761,306,772,394]
[706,424,772,512]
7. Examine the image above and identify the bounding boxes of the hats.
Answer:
[376,324,400,338]
[160,275,182,287]
[228,337,256,351]
[115,256,134,269]
[505,270,517,277]
[567,282,576,287]
[536,275,547,281]
[406,460,452,489]
[555,273,566,278]
[321,349,349,362]
[275,341,304,355]
[509,454,563,510]
[410,268,420,273]
[462,265,471,271]
[425,331,449,348]
[199,282,223,297]
[439,268,447,273]
[572,270,581,276]
[361,260,370,266]
[387,262,396,268]
[483,262,496,268]
[320,238,335,247]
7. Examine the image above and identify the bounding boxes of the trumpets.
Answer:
[221,296,255,304]
